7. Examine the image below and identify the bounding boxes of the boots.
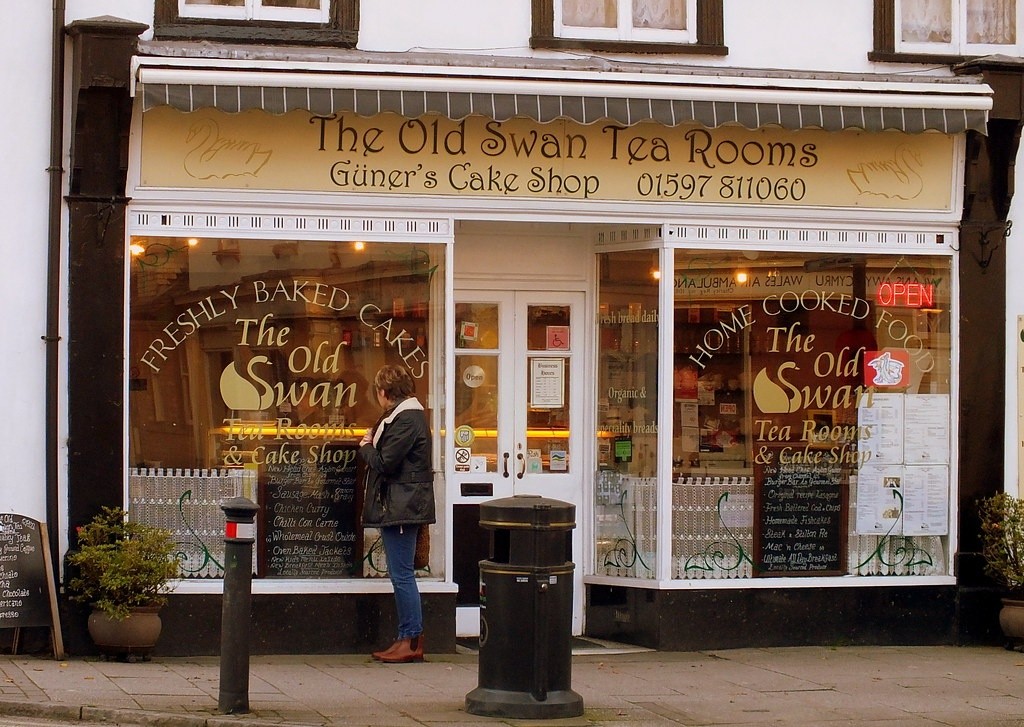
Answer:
[379,634,423,663]
[372,638,403,659]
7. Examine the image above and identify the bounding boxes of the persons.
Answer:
[359,365,436,662]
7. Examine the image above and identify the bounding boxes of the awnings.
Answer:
[137,68,987,135]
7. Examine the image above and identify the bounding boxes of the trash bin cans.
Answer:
[476,493,577,693]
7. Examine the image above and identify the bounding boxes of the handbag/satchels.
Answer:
[415,522,429,569]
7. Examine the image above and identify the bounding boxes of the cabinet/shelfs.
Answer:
[176,271,756,474]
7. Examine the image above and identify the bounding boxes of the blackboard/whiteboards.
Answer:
[751,440,849,579]
[0,511,54,630]
[255,439,366,578]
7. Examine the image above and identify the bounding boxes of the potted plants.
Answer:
[974,491,1023,649]
[66,506,182,663]
